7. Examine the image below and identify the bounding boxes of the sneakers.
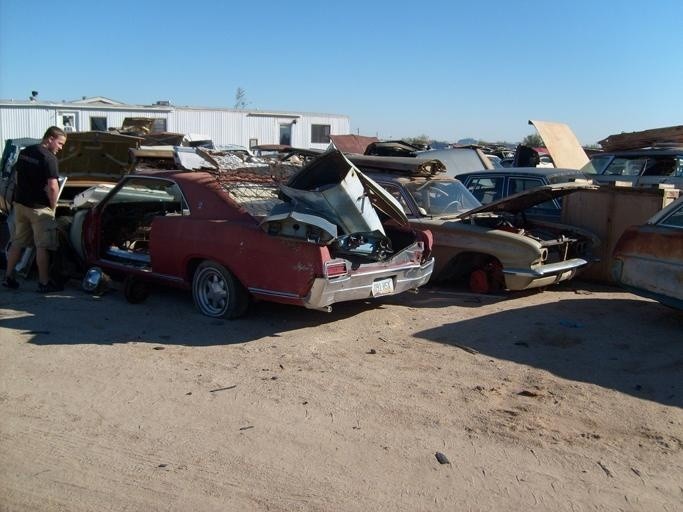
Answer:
[2,275,20,289]
[36,281,63,294]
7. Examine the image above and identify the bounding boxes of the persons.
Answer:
[2,126,67,293]
